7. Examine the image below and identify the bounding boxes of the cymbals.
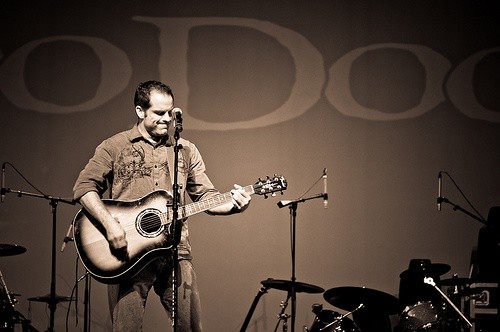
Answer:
[26,293,77,304]
[259,279,325,295]
[439,273,470,287]
[323,285,402,315]
[0,243,28,257]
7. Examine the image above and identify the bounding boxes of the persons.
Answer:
[72,80,251,332]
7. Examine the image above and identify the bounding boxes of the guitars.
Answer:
[71,173,288,285]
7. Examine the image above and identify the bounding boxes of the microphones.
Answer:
[437,172,442,211]
[323,168,328,208]
[172,107,183,132]
[1,164,6,203]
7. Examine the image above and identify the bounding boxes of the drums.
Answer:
[397,298,457,332]
[307,309,359,332]
